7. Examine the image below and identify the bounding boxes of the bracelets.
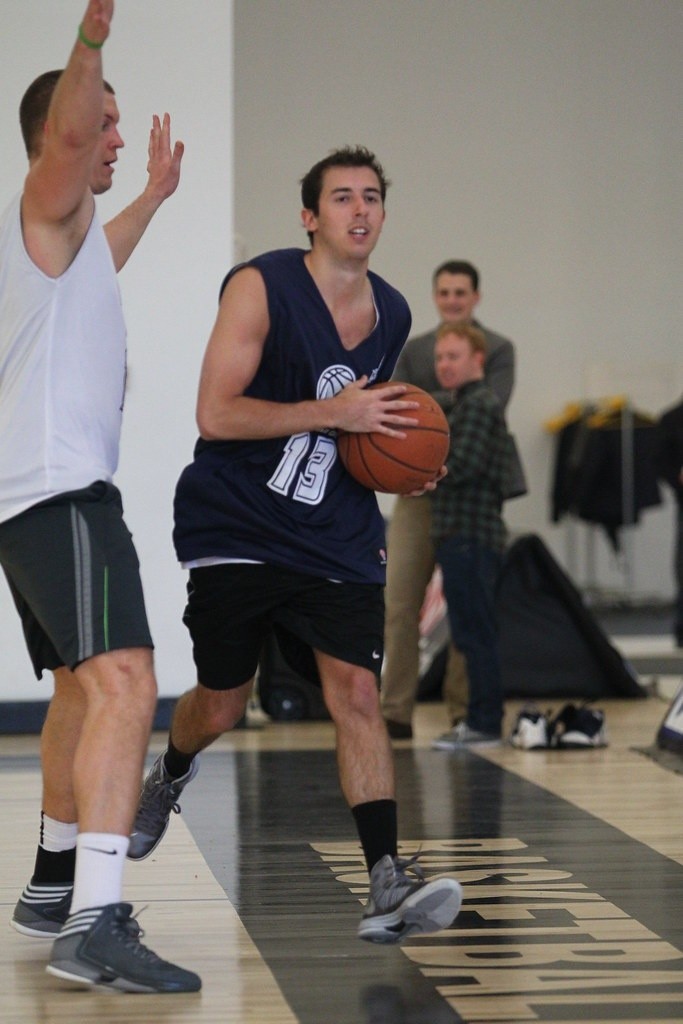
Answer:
[78,21,103,49]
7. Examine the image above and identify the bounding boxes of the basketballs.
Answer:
[338,381,450,493]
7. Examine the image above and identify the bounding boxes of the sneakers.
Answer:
[510,705,611,750]
[357,843,463,946]
[430,722,505,750]
[385,719,413,749]
[10,876,140,938]
[125,748,201,862]
[44,902,201,993]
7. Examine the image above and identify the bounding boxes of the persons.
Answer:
[123,148,464,948]
[0,1,206,999]
[427,323,507,752]
[377,258,527,741]
